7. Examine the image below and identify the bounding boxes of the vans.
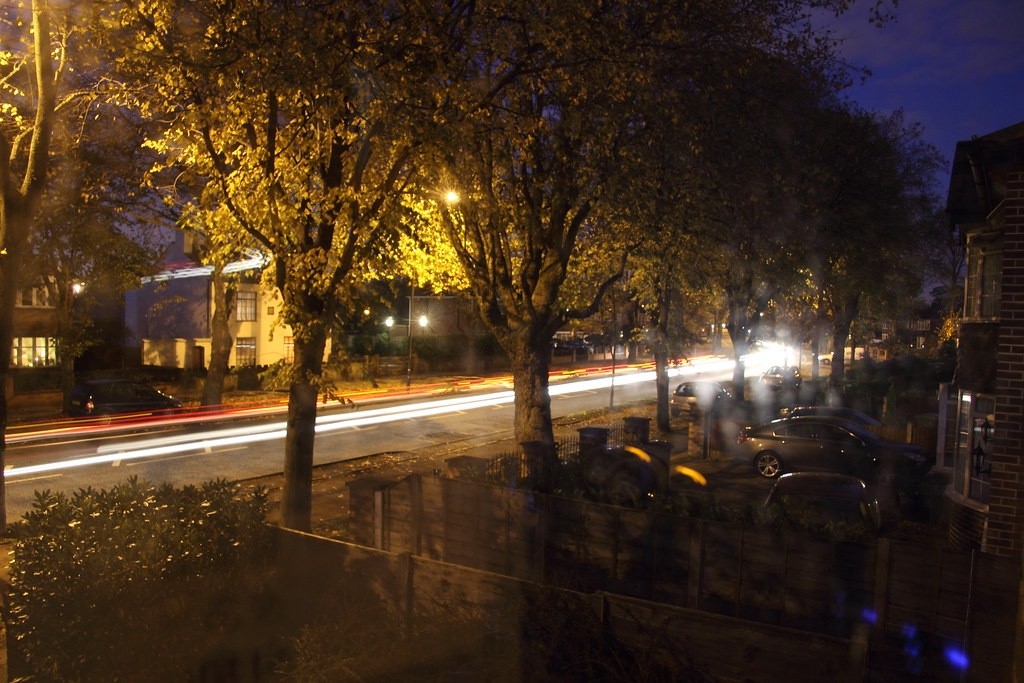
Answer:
[67,379,182,426]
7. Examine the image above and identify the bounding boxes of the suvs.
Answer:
[818,347,866,367]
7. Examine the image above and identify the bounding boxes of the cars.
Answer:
[760,365,802,391]
[732,414,930,491]
[754,471,884,541]
[774,405,880,441]
[673,383,737,416]
